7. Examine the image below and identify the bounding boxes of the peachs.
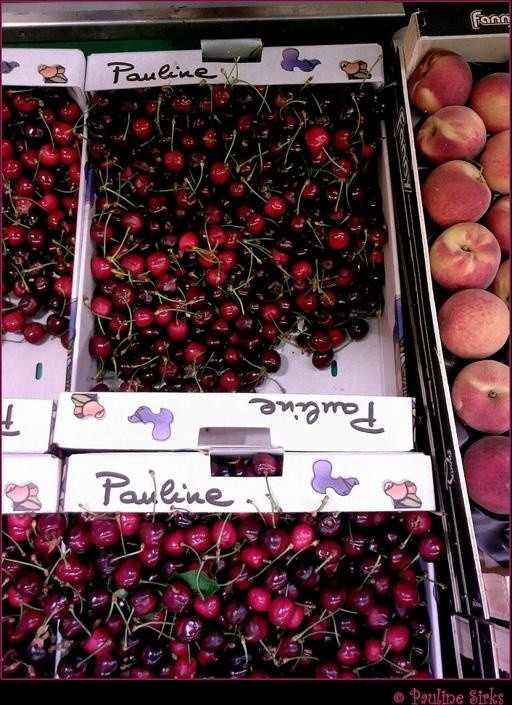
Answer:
[408,47,511,518]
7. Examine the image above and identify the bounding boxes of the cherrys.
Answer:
[1,83,446,679]
